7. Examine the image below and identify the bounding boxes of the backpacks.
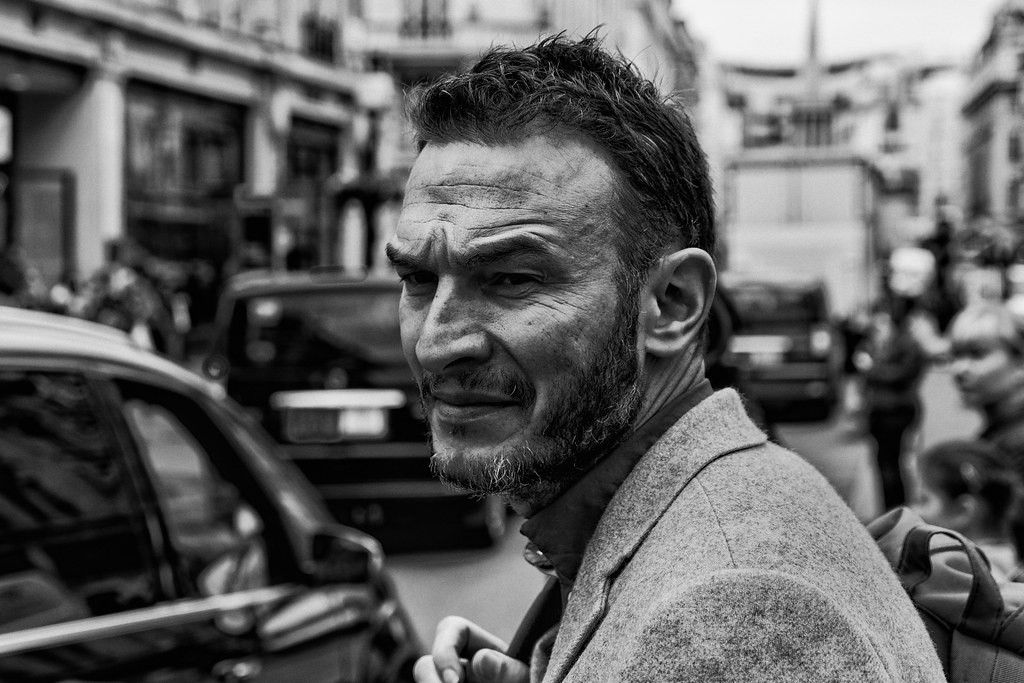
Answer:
[505,506,1024,683]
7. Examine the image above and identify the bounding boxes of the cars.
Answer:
[726,284,847,419]
[1,301,435,683]
[219,263,513,546]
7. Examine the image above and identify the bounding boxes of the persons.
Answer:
[856,204,1024,586]
[386,25,945,683]
[0,240,187,360]
